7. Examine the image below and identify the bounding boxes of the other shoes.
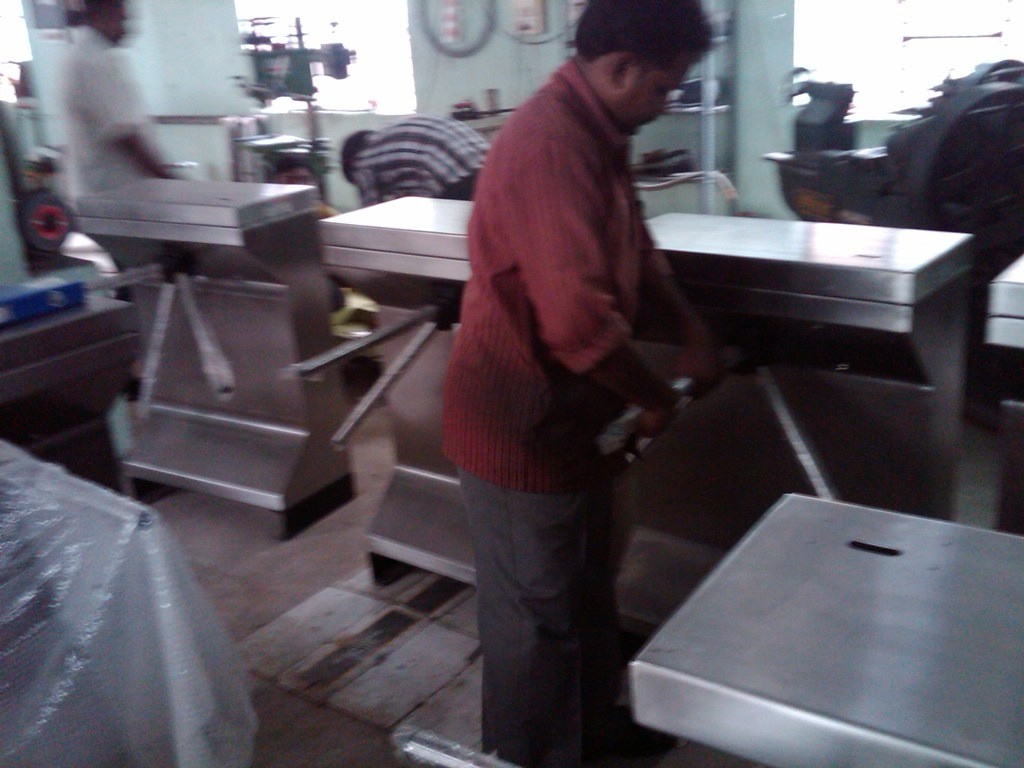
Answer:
[493,705,672,768]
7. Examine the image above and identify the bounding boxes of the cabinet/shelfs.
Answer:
[559,27,743,218]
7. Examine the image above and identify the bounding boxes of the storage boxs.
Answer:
[0,278,89,326]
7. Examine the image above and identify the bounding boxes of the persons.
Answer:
[266,158,341,221]
[47,0,190,406]
[344,112,492,330]
[436,0,720,768]
